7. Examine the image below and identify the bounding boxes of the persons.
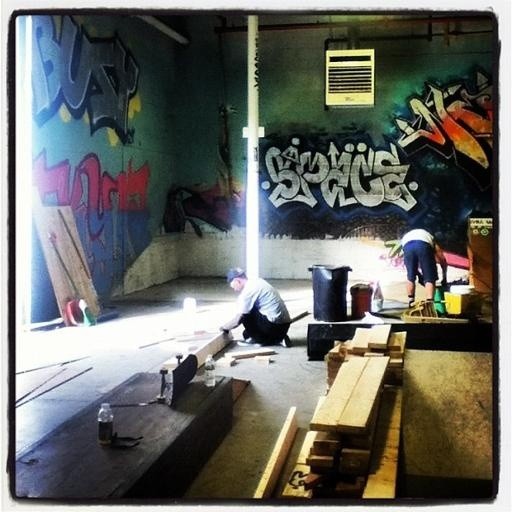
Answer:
[401,228,448,309]
[219,268,291,347]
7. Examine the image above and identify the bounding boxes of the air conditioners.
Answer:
[324,48,376,109]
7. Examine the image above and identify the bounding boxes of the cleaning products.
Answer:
[78,296,96,327]
[66,297,83,326]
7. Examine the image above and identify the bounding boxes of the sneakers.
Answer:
[279,336,291,347]
[237,337,262,348]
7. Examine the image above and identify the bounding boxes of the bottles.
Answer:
[97,403,114,446]
[204,354,216,387]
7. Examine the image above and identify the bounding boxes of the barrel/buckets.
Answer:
[350,284,374,319]
[308,262,353,323]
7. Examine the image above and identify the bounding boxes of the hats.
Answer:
[227,267,246,282]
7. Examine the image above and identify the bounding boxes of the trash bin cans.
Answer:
[308,264,373,321]
